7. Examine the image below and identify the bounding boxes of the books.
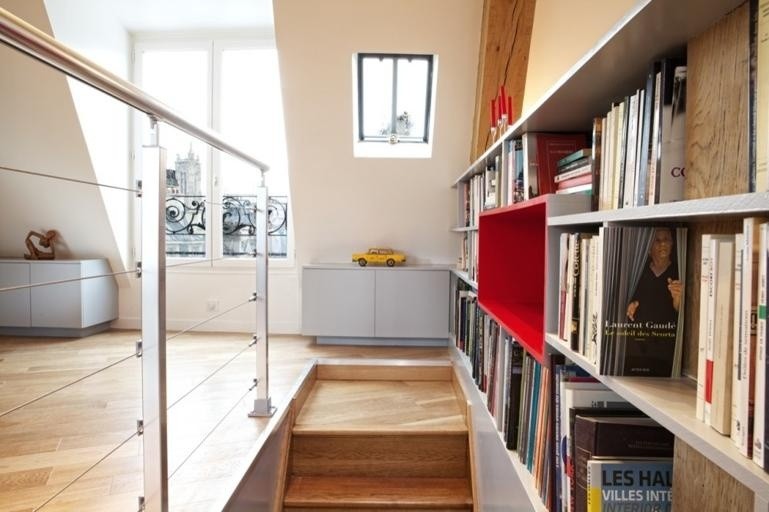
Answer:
[521,66,769,474]
[455,140,524,282]
[456,279,675,512]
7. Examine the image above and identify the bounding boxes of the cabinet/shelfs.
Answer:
[448,0,768,511]
[1,255,119,339]
[299,263,452,348]
